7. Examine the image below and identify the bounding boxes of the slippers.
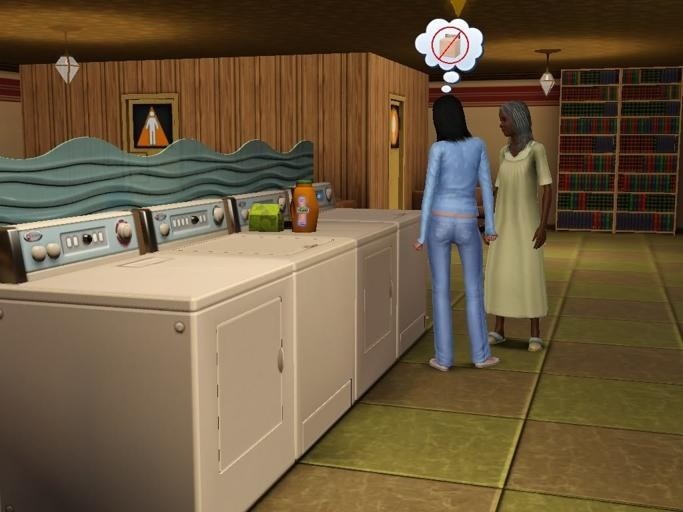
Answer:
[527,338,544,352]
[487,332,505,345]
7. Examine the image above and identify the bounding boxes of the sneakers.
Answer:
[429,358,448,373]
[475,357,499,369]
